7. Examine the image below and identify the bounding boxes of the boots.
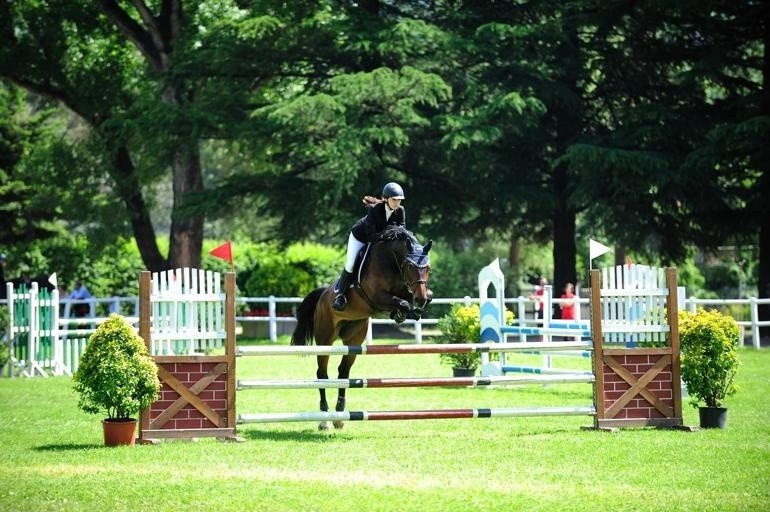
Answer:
[332,267,354,312]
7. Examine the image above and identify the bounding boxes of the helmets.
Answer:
[382,182,406,200]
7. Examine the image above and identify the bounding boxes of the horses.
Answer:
[289,225,433,430]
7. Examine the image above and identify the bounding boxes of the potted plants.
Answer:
[72,310,163,452]
[432,303,485,379]
[677,304,745,430]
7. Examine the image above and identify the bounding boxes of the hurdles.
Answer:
[234,339,597,424]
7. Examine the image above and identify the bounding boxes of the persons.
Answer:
[557,282,576,342]
[57,282,70,330]
[331,179,407,311]
[29,273,58,291]
[69,281,93,329]
[530,276,550,327]
[13,270,33,290]
